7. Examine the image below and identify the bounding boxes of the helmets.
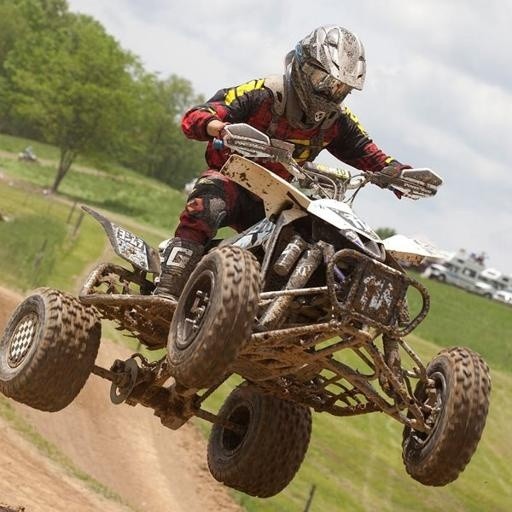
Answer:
[289,25,366,126]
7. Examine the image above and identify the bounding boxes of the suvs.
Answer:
[0,132,497,497]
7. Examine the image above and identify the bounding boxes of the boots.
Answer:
[154,240,205,300]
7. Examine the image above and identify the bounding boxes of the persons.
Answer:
[136,25,430,346]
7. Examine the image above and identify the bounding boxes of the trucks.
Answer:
[427,248,511,305]
